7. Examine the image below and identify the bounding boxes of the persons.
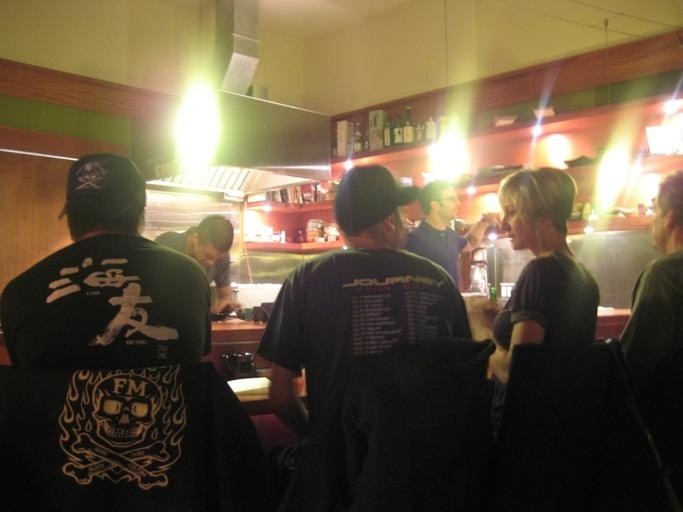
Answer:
[400,180,502,287]
[483,167,600,372]
[255,163,477,511]
[0,153,213,362]
[602,168,683,512]
[152,214,242,320]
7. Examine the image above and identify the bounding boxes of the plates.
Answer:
[561,157,606,167]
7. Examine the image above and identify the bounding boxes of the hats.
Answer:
[334,165,421,234]
[56,152,148,220]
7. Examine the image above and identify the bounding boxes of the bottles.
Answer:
[351,107,463,156]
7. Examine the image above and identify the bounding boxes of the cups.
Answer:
[221,352,257,378]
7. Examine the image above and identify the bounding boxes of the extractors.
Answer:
[137,1,335,203]
